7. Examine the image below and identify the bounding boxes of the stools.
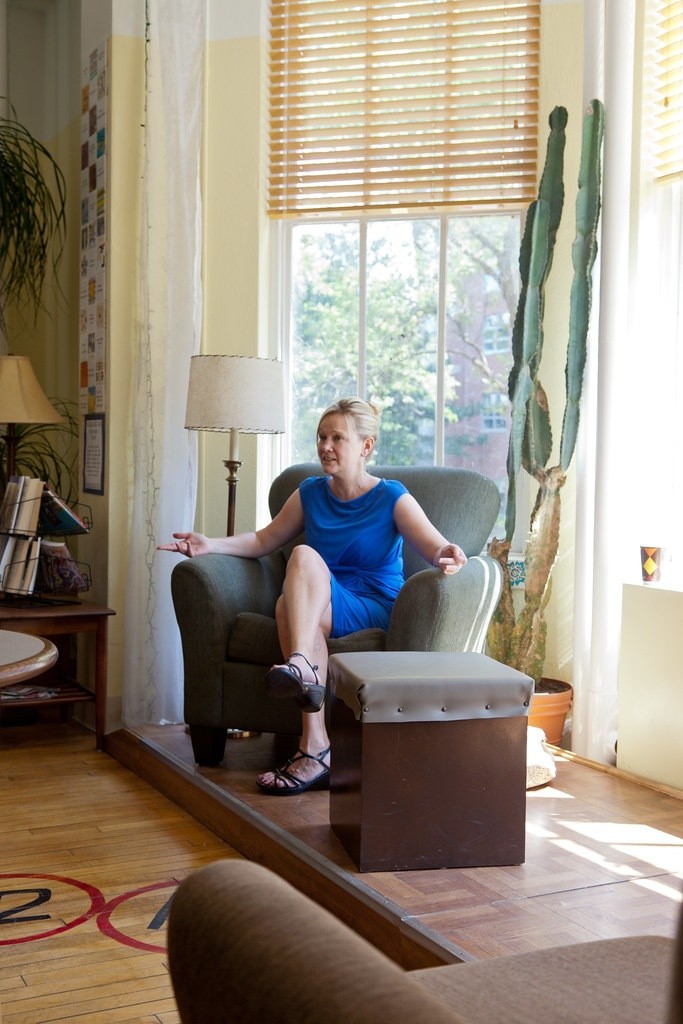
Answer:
[326,651,535,872]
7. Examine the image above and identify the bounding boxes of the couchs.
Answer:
[166,858,683,1024]
[170,463,504,767]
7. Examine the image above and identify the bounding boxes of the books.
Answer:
[0,476,89,595]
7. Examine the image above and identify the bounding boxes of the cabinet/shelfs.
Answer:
[1,476,94,607]
[0,605,118,751]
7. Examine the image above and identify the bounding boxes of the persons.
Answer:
[156,398,467,796]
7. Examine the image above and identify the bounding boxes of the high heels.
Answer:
[265,651,325,713]
[256,746,336,794]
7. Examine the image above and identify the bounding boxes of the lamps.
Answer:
[0,353,65,497]
[183,355,287,740]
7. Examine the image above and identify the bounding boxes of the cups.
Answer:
[641,546,661,585]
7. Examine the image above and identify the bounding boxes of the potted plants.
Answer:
[487,99,606,748]
